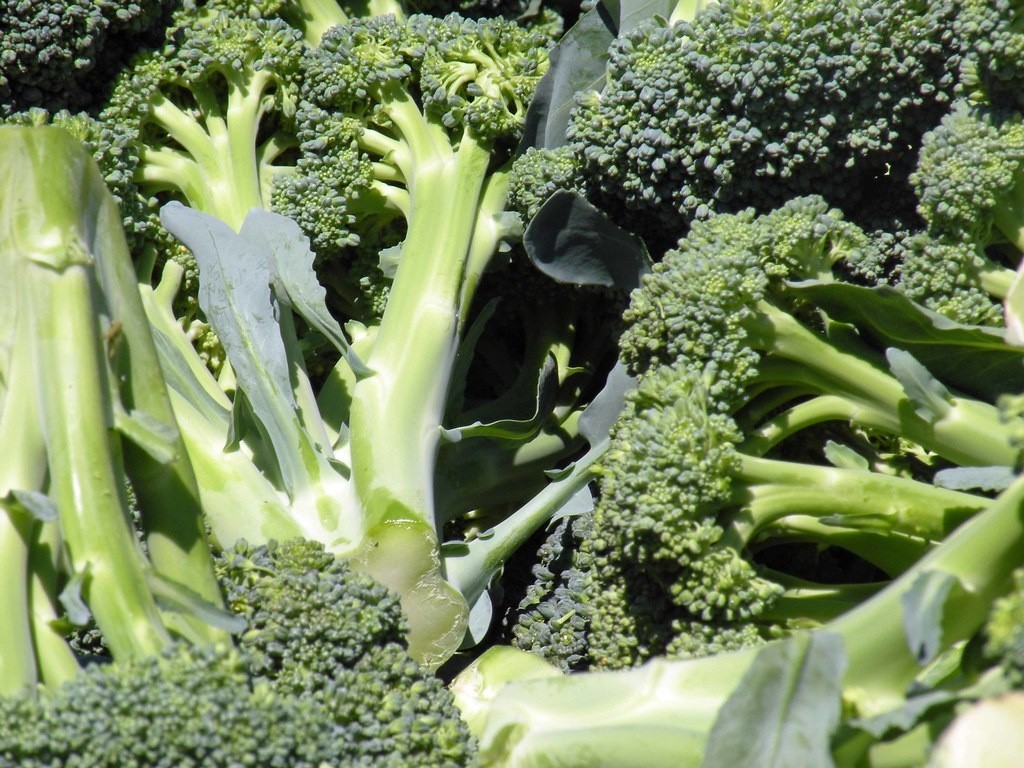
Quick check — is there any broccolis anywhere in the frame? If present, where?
[0,0,1024,768]
